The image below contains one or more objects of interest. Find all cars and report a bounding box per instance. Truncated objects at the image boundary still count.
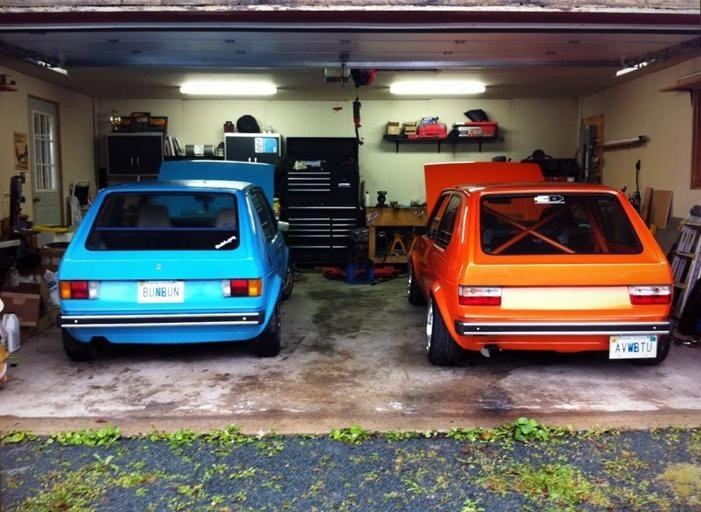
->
[407,159,679,367]
[54,160,298,359]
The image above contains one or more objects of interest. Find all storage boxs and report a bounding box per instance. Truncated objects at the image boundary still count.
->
[1,278,51,328]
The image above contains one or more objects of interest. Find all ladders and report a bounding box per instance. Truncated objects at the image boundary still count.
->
[668,215,701,328]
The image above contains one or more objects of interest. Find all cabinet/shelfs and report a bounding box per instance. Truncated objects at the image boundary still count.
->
[105,131,369,275]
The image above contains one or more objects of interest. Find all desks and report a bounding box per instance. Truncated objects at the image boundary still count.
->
[364,203,434,268]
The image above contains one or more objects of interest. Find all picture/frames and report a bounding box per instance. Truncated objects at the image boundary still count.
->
[116,110,169,135]
